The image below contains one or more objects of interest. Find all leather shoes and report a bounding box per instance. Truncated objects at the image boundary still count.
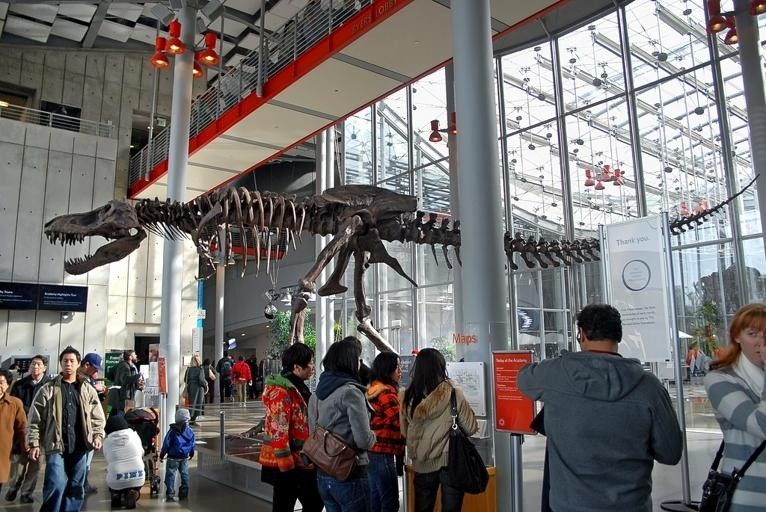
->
[4,488,34,503]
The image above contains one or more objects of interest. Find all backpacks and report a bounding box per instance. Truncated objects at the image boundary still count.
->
[107,361,131,381]
[220,359,232,376]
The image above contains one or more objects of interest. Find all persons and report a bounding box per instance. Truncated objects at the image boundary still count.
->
[703,303,766,512]
[184,350,262,425]
[400,348,478,512]
[517,304,683,512]
[1,345,145,512]
[158,409,195,503]
[258,343,324,512]
[308,336,403,512]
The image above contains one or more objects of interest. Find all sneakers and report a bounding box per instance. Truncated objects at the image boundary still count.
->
[111,490,137,510]
[166,492,188,500]
[192,421,200,427]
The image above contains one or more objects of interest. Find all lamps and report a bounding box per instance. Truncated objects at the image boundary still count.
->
[584,165,624,191]
[707,1,766,44]
[678,201,706,217]
[429,112,457,142]
[150,22,219,78]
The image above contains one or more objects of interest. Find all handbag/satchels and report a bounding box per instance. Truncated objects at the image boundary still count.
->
[699,468,738,512]
[441,424,489,494]
[208,364,216,381]
[182,384,189,399]
[234,372,241,378]
[302,425,356,481]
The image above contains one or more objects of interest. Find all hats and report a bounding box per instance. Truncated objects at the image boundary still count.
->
[175,409,190,423]
[83,353,101,369]
